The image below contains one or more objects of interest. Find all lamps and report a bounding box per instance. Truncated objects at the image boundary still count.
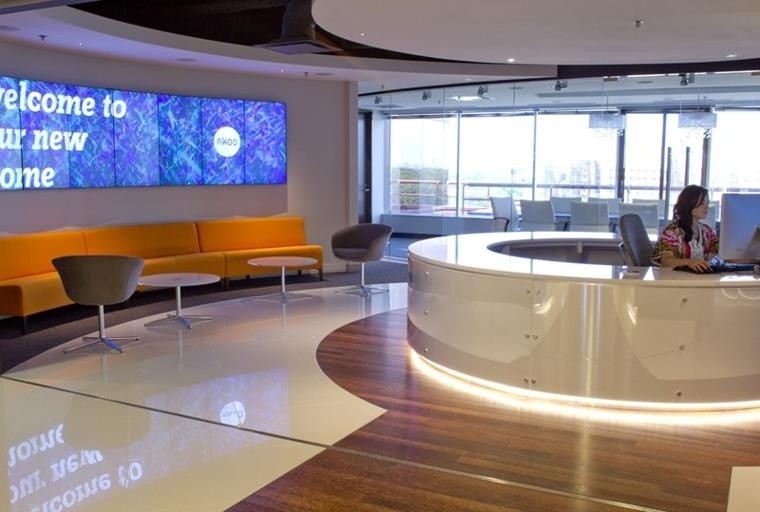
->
[552,79,571,93]
[589,80,625,137]
[476,83,490,98]
[677,74,697,87]
[421,89,432,102]
[678,94,716,139]
[372,95,384,104]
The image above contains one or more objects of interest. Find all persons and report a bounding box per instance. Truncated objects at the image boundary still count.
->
[649,185,718,274]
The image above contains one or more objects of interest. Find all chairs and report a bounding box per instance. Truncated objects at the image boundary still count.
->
[633,199,665,216]
[332,223,392,296]
[617,213,654,267]
[520,200,569,231]
[619,203,663,232]
[588,197,622,215]
[550,197,581,212]
[51,256,144,354]
[570,200,616,232]
[490,196,520,232]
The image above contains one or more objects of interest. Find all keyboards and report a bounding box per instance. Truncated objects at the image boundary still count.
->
[710,264,755,272]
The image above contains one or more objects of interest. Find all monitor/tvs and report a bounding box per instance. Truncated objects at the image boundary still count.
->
[718,193,760,275]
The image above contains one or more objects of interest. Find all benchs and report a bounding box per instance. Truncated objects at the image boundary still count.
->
[0,216,324,334]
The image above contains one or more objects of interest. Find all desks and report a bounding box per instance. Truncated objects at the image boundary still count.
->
[518,213,671,232]
